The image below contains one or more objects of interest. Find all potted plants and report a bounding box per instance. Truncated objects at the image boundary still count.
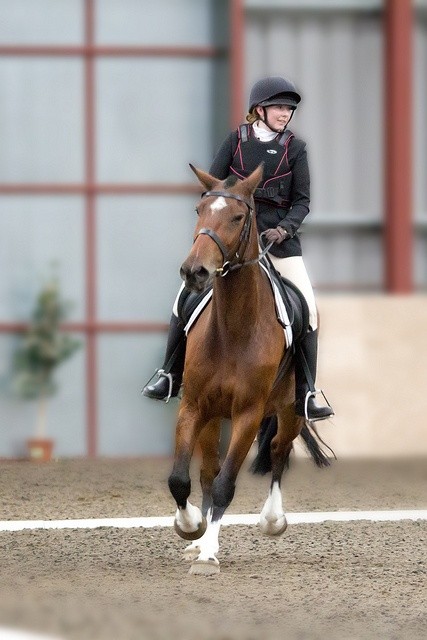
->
[9,282,83,464]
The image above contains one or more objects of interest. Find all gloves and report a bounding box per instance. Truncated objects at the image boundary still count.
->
[265,226,288,247]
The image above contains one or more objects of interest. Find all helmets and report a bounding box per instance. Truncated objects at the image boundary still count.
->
[249,77,301,114]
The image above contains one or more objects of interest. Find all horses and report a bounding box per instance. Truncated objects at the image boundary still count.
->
[167,159,336,576]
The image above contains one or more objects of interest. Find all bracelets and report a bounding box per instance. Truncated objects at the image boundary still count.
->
[276,225,286,238]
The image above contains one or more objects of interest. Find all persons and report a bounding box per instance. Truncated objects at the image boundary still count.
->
[141,77,334,420]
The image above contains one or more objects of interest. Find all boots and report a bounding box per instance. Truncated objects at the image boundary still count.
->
[295,329,333,417]
[143,312,186,399]
